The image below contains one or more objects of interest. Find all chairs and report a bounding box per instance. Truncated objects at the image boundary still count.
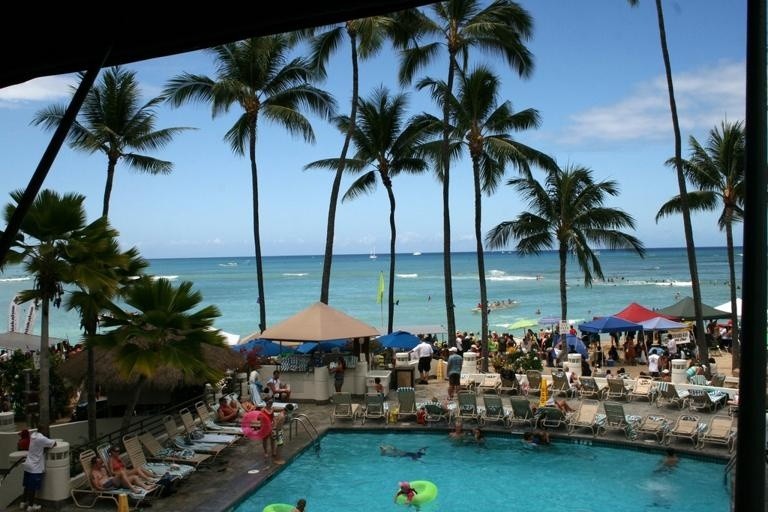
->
[209,402,263,431]
[123,435,193,483]
[98,444,162,497]
[248,387,298,420]
[140,429,209,472]
[70,449,149,506]
[220,393,283,434]
[178,407,241,450]
[193,400,252,441]
[163,416,228,460]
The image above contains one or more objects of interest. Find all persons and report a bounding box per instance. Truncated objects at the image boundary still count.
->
[373,321,740,395]
[523,399,576,449]
[417,396,491,449]
[216,370,295,464]
[291,499,305,512]
[326,356,345,392]
[19,422,57,512]
[477,297,514,314]
[380,443,431,465]
[90,457,157,494]
[107,445,158,483]
[393,479,420,512]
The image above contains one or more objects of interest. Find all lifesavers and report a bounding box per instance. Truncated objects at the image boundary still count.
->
[265,504,296,512]
[396,481,438,506]
[242,412,272,441]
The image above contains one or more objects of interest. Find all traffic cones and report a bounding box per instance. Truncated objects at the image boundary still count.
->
[118,493,129,512]
[539,378,548,406]
[436,358,445,381]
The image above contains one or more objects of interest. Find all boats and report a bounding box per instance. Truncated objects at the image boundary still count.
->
[471,296,521,317]
